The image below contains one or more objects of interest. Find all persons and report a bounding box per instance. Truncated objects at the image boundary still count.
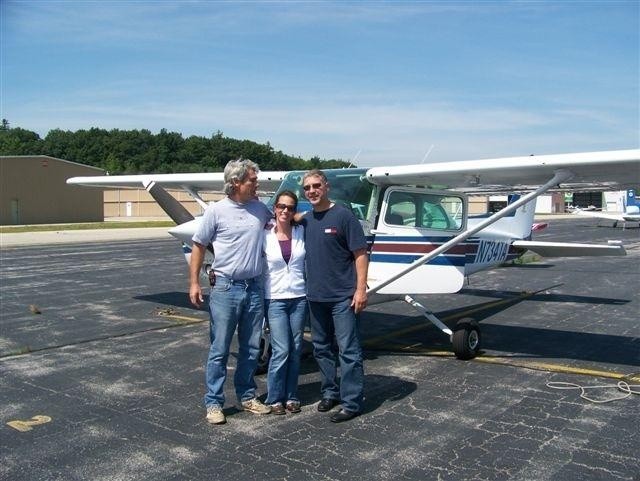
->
[304,169,369,423]
[189,158,277,425]
[258,190,307,416]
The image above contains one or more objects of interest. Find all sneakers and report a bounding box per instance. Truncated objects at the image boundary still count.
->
[236,397,271,414]
[206,406,226,424]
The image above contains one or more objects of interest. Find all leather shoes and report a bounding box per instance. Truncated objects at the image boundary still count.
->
[270,402,286,415]
[317,399,336,411]
[286,401,302,413]
[331,408,357,423]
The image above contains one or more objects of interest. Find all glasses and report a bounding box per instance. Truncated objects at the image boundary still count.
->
[275,204,296,212]
[303,184,321,190]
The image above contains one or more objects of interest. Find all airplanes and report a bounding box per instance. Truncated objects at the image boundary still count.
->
[65,147,639,374]
[573,190,639,230]
